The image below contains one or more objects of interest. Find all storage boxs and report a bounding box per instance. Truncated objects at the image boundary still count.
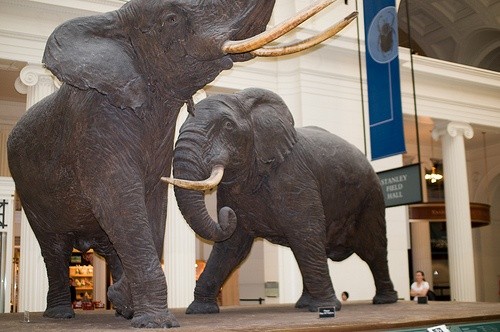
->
[73,301,104,311]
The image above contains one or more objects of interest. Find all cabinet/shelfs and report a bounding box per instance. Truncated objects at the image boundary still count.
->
[72,275,94,300]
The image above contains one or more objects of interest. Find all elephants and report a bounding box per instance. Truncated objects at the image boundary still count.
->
[160,87,398,314]
[6,0,359,327]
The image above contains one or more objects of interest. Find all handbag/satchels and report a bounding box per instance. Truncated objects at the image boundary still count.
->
[428,290,436,301]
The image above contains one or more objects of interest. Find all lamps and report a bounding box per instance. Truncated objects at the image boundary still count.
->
[424,129,443,183]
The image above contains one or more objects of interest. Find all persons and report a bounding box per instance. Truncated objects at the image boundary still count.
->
[342,291,349,301]
[69,277,76,305]
[410,271,429,301]
